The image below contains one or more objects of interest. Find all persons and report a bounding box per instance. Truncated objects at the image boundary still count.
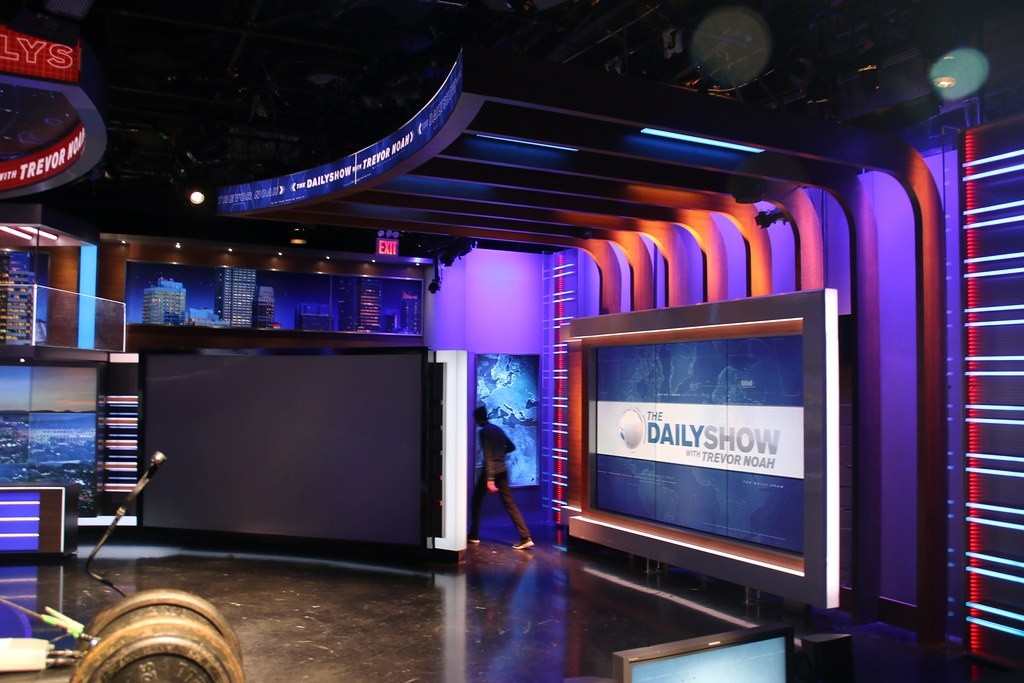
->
[470,407,533,550]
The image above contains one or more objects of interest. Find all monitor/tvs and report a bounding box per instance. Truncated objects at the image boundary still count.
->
[611,620,795,683]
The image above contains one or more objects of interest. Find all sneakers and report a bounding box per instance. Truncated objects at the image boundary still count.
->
[468,534,480,543]
[512,538,535,550]
